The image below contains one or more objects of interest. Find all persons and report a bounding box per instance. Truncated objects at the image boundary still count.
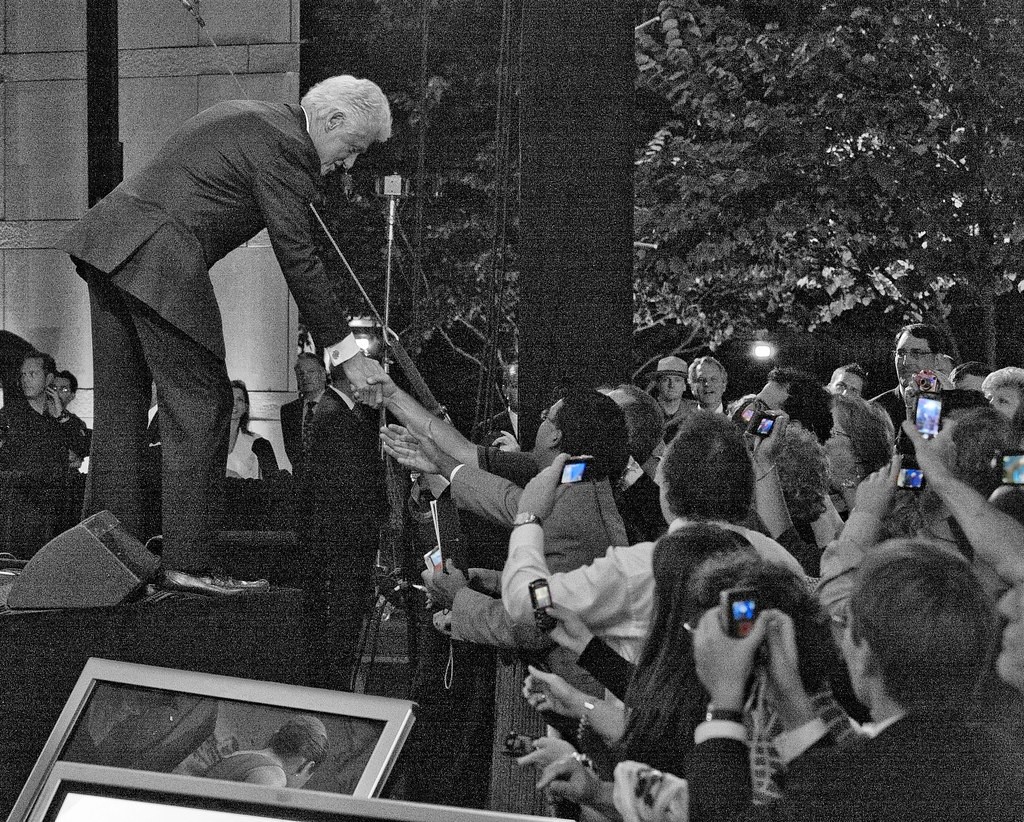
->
[203,716,327,788]
[0,350,92,559]
[350,325,1024,822]
[57,75,383,595]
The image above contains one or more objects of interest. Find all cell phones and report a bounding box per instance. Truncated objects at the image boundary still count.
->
[914,391,943,441]
[898,468,927,492]
[528,578,557,631]
[559,458,597,484]
[996,450,1024,486]
[720,588,758,639]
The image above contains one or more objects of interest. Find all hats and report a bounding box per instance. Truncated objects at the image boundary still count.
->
[648,355,689,379]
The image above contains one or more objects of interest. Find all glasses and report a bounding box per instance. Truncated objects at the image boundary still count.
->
[890,349,937,359]
[50,384,70,395]
[829,427,850,440]
[540,409,556,425]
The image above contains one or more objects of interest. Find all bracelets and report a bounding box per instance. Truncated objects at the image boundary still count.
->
[755,462,778,482]
[569,752,597,772]
[577,697,596,742]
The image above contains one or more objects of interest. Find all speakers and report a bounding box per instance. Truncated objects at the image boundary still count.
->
[5,510,162,608]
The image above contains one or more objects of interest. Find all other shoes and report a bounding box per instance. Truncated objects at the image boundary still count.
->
[161,567,270,597]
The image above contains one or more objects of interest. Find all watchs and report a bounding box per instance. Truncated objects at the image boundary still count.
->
[55,410,69,423]
[839,476,855,496]
[512,512,542,527]
[705,703,744,724]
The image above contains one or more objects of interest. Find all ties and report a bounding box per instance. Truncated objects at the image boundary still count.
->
[302,401,316,425]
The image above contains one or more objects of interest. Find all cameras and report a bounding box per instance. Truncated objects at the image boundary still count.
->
[747,409,777,440]
[423,546,441,572]
[920,374,941,393]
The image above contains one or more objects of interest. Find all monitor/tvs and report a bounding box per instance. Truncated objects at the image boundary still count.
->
[7,656,577,822]
